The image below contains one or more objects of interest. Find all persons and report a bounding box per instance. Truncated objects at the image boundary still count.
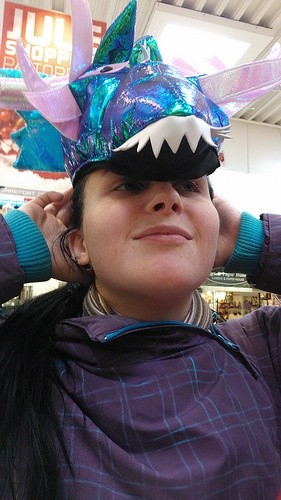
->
[1,0,281,500]
